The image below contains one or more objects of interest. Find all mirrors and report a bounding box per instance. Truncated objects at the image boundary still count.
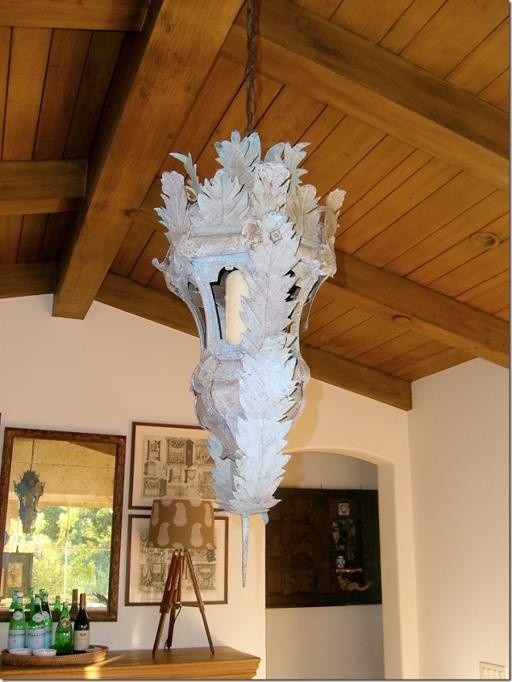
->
[0,427,127,622]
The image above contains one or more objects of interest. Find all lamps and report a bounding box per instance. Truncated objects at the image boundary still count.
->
[150,0,349,590]
[12,438,45,538]
[146,499,217,660]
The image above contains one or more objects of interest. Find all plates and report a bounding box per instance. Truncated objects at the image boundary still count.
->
[8,647,57,659]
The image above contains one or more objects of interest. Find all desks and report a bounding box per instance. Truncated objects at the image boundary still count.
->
[0,645,261,680]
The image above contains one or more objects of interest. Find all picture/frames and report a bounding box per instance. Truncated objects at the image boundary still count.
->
[127,421,225,512]
[2,552,33,598]
[125,513,228,607]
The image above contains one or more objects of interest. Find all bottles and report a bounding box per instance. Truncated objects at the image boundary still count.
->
[9,587,80,613]
[7,591,90,654]
